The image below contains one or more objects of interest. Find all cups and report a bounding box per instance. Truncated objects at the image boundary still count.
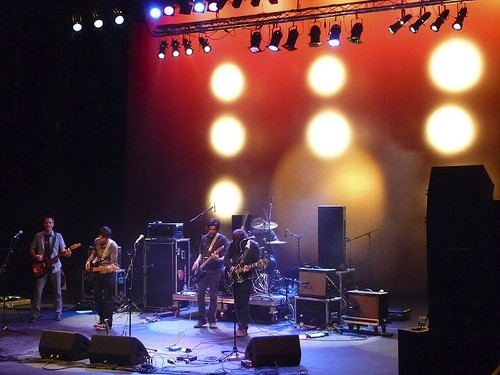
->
[419,317,427,330]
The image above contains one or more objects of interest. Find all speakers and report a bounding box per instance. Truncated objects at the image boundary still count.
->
[39,329,150,366]
[298,268,336,299]
[245,335,301,367]
[347,290,388,319]
[317,205,347,271]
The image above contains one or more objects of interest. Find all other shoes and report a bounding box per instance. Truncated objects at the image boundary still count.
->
[94,321,111,330]
[31,317,37,322]
[56,315,61,321]
[194,321,206,328]
[211,322,217,328]
[235,327,247,338]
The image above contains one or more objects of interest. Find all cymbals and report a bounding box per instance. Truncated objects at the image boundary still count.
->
[266,240,287,245]
[252,221,278,229]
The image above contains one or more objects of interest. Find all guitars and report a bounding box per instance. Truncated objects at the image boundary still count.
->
[225,258,267,287]
[190,245,224,283]
[31,243,81,279]
[82,264,120,281]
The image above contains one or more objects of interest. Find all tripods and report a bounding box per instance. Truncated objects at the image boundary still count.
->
[219,240,245,362]
[270,277,303,328]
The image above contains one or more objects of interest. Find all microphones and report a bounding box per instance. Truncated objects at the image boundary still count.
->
[14,230,23,238]
[284,229,288,237]
[243,236,256,241]
[303,281,309,284]
[142,264,154,268]
[349,305,358,308]
[270,197,272,208]
[214,203,215,212]
[134,234,144,243]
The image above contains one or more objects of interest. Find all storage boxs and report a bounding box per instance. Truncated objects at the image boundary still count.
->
[82,246,127,298]
[295,268,411,325]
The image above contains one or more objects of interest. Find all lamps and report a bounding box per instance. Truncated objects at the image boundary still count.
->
[72,0,469,59]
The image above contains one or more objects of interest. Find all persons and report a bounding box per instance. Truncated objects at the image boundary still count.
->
[29,215,72,323]
[193,218,228,329]
[85,226,119,330]
[249,220,279,292]
[224,228,260,337]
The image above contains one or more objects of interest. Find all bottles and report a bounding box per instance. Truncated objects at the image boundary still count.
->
[183,284,187,292]
